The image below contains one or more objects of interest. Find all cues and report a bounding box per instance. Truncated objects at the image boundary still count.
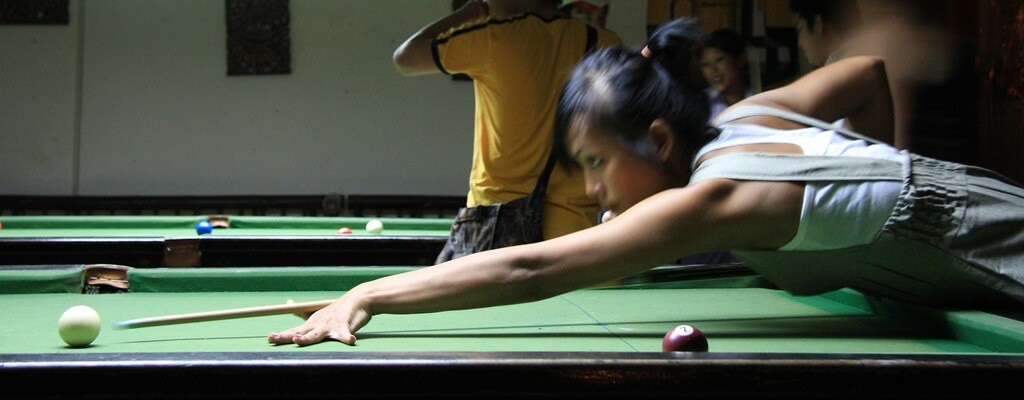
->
[113,264,753,331]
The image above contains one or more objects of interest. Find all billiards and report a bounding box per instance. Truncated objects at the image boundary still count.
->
[336,227,353,234]
[365,219,383,234]
[57,304,101,347]
[662,324,709,353]
[196,221,212,235]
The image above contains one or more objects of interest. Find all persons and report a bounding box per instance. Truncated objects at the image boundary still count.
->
[694,28,759,126]
[789,0,920,161]
[267,16,1024,346]
[392,0,625,288]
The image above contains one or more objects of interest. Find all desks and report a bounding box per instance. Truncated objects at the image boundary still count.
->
[0,215,456,265]
[0,263,1024,400]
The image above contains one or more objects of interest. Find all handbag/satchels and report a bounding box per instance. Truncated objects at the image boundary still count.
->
[450,197,544,265]
